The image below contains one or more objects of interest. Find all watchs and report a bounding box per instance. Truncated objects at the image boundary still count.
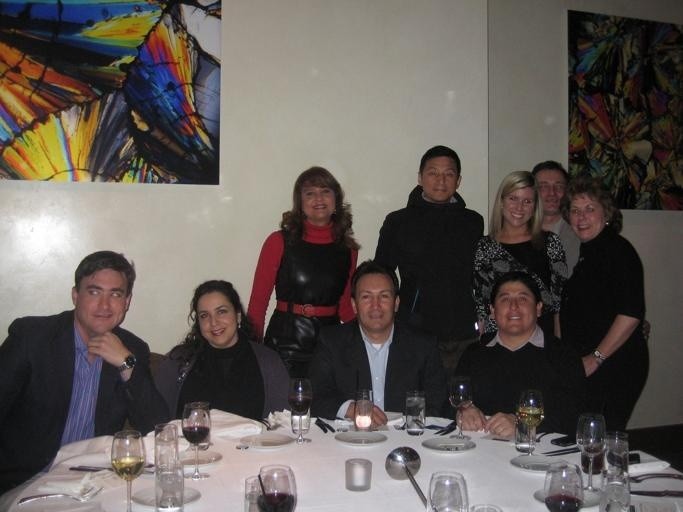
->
[116,352,138,373]
[593,349,609,361]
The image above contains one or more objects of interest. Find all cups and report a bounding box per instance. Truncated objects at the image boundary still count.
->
[447,376,472,439]
[183,402,210,478]
[545,462,583,512]
[354,389,373,430]
[518,391,546,457]
[427,471,466,512]
[289,397,310,436]
[154,467,185,510]
[575,416,603,491]
[258,465,297,512]
[407,392,425,436]
[345,458,372,492]
[598,484,632,512]
[603,431,632,496]
[110,431,146,512]
[288,379,310,445]
[513,412,540,453]
[244,477,261,511]
[154,424,178,484]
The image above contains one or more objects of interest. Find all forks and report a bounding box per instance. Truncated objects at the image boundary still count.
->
[17,486,106,506]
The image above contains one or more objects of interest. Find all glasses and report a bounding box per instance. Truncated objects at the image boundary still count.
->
[503,194,534,206]
[569,204,598,215]
[538,184,564,192]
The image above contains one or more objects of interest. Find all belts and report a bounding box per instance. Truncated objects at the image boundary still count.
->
[275,300,338,318]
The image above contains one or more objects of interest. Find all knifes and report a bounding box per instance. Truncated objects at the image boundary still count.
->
[631,490,683,498]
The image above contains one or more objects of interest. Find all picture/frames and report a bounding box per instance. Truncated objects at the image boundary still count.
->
[568,9,683,212]
[0,0,223,185]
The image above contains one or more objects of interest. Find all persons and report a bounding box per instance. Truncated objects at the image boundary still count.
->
[246,166,360,379]
[559,176,651,440]
[373,145,485,418]
[472,170,569,342]
[530,160,582,341]
[0,250,172,496]
[164,279,292,423]
[308,261,448,432]
[448,270,586,442]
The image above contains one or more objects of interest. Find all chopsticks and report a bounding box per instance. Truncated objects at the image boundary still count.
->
[70,465,154,478]
[544,447,577,456]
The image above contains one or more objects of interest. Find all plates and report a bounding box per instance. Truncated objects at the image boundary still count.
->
[533,486,603,507]
[334,432,387,445]
[511,455,567,472]
[177,449,222,466]
[240,433,293,448]
[132,486,202,507]
[421,438,476,452]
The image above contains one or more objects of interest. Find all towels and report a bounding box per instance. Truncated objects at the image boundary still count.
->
[147,407,263,441]
[603,447,671,476]
